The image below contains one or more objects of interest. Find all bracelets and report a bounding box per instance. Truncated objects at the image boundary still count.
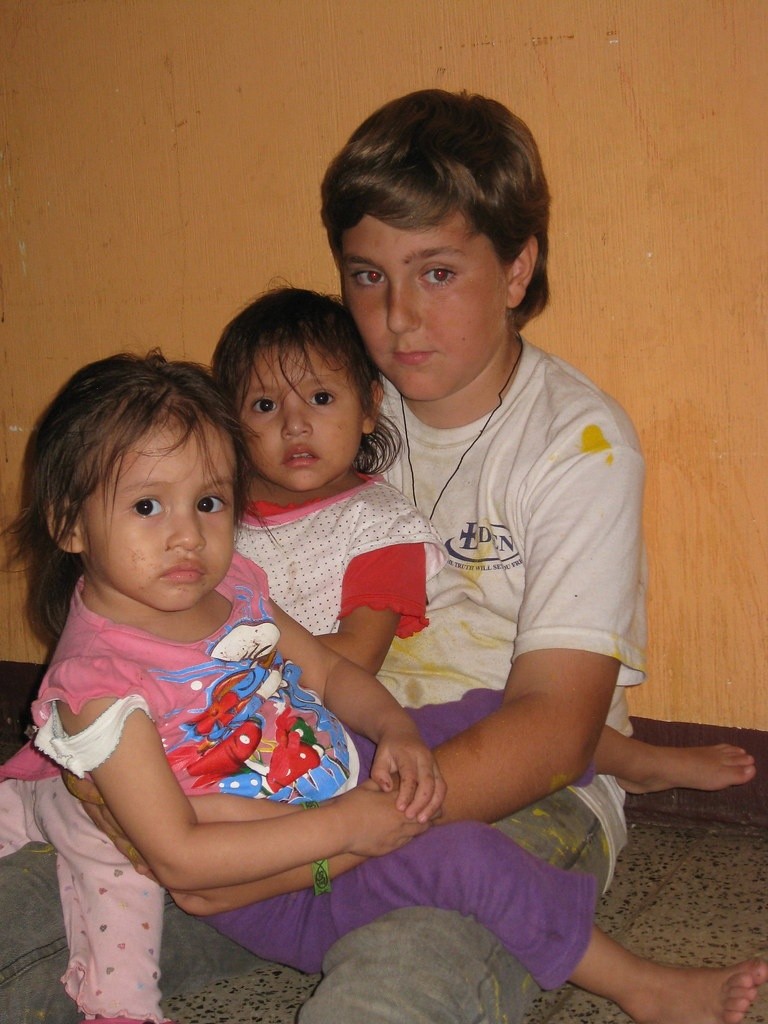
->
[302,799,333,895]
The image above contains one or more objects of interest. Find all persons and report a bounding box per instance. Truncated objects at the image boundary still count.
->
[1,87,649,1024]
[2,348,767,1022]
[2,282,431,1024]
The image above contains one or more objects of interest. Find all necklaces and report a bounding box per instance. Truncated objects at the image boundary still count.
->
[395,330,525,521]
